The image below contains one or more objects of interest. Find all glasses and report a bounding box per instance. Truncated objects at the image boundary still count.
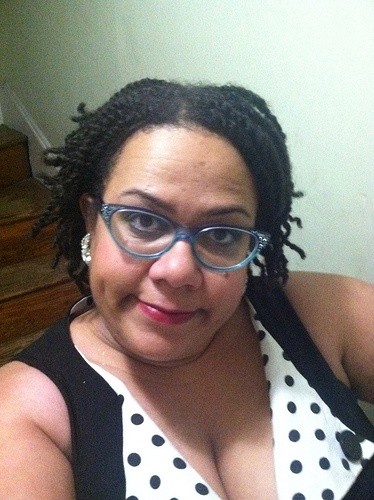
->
[91,194,269,273]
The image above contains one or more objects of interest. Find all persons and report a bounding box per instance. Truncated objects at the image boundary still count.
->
[2,76,373,499]
